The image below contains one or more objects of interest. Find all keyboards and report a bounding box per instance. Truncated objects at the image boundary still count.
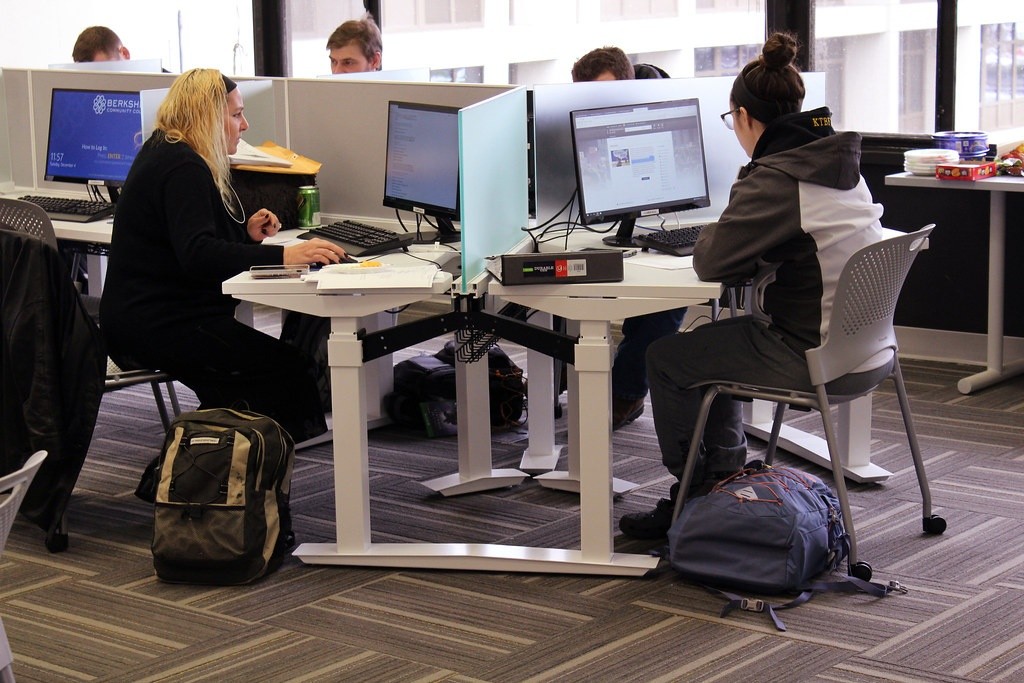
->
[296,220,415,257]
[634,225,707,258]
[16,195,116,223]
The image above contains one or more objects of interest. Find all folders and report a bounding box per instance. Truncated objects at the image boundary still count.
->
[484,250,624,287]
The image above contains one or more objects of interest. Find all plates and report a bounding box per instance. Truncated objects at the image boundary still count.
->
[904,149,959,175]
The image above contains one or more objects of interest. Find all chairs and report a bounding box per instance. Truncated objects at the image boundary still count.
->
[0,449,49,682]
[0,199,183,552]
[670,224,947,584]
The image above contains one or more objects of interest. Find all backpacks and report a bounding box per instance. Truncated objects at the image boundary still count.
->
[136,404,295,590]
[665,461,848,613]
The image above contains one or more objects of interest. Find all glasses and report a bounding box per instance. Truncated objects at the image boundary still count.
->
[720,107,743,131]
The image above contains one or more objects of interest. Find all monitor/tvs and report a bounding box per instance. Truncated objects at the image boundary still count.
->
[382,100,461,244]
[44,88,144,204]
[569,97,711,248]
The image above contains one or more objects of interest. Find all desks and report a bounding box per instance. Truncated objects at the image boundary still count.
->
[885,170,1024,394]
[2,184,892,580]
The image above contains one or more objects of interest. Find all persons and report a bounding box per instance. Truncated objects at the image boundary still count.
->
[326,19,382,73]
[72,26,130,295]
[522,47,685,424]
[98,69,349,502]
[621,32,895,538]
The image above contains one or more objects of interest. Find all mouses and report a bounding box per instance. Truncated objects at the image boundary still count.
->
[312,255,358,266]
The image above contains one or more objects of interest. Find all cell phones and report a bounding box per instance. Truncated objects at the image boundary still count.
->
[250,264,310,276]
[579,247,636,258]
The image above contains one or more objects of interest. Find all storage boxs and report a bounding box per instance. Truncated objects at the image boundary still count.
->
[936,159,997,180]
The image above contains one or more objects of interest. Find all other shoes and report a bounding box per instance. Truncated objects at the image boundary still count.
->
[612,400,645,428]
[618,484,687,541]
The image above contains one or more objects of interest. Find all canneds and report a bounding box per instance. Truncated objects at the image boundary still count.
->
[298,185,321,230]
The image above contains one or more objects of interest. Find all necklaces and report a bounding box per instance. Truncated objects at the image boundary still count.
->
[224,184,245,224]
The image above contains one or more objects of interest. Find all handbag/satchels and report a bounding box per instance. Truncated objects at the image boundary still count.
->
[387,341,525,438]
